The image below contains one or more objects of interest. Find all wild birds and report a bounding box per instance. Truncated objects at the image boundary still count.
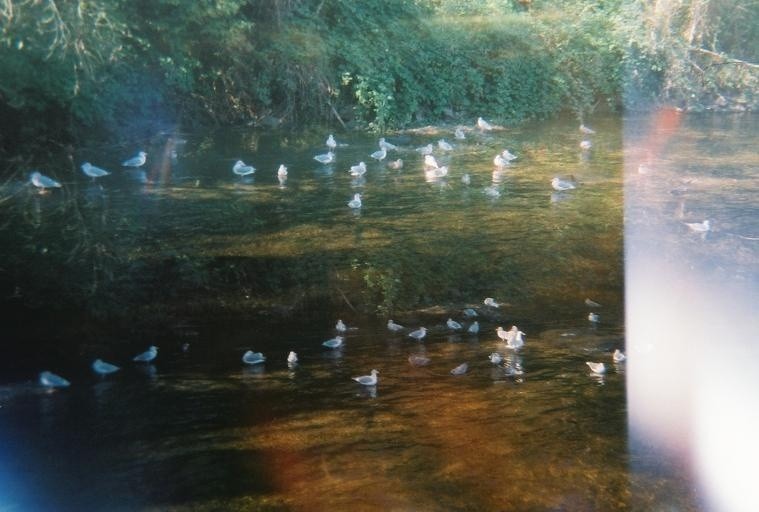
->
[232,117,595,209]
[80,163,112,177]
[134,345,159,361]
[93,359,120,375]
[122,151,148,167]
[39,371,70,394]
[30,171,61,195]
[638,164,651,177]
[685,220,711,232]
[242,297,626,385]
